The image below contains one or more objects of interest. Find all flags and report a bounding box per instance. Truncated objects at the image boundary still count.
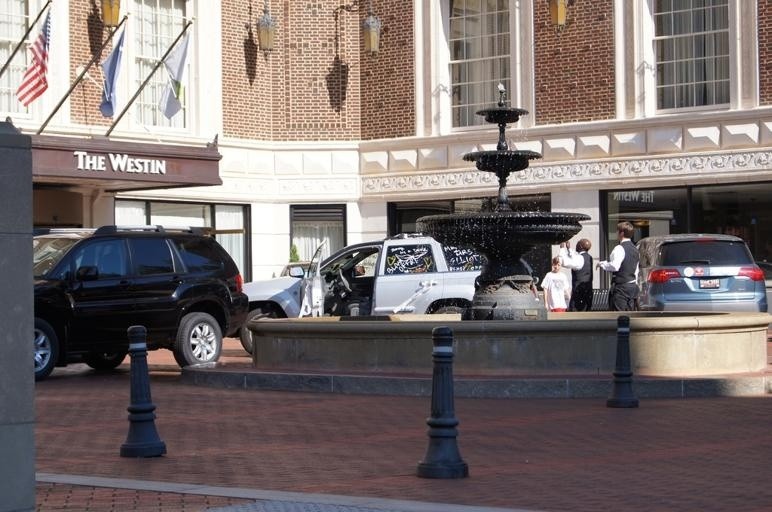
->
[158,26,191,120]
[15,8,51,107]
[99,24,126,117]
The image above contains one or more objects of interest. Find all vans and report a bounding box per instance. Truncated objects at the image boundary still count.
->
[632,230,770,316]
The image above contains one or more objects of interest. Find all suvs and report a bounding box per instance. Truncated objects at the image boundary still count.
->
[33,225,252,382]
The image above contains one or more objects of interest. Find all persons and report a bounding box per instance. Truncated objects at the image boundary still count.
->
[595,222,640,311]
[541,239,593,311]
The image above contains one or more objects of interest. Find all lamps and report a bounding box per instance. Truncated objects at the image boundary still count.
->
[548,0,575,24]
[331,0,382,54]
[245,1,273,50]
[89,0,119,28]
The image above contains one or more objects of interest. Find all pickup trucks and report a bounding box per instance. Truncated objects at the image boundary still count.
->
[241,229,481,356]
